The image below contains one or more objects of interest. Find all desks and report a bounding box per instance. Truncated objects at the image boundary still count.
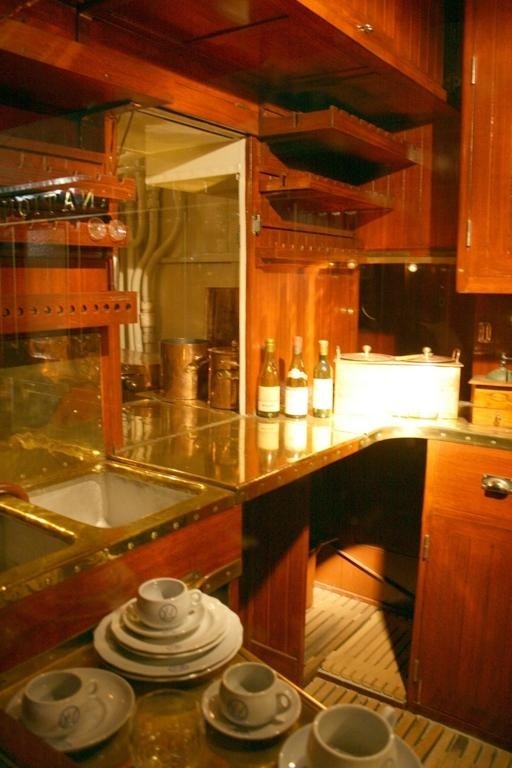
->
[0,640,374,767]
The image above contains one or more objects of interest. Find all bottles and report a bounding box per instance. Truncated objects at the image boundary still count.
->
[256,419,279,475]
[310,421,332,454]
[286,335,309,419]
[313,340,333,419]
[284,421,307,466]
[256,337,281,418]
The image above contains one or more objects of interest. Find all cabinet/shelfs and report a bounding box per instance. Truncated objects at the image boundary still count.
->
[254,107,415,417]
[453,2,510,296]
[0,476,311,739]
[406,421,511,754]
[131,1,448,130]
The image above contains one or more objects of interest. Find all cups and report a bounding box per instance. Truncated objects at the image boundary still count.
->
[308,703,398,768]
[22,670,99,739]
[220,662,294,727]
[137,577,203,629]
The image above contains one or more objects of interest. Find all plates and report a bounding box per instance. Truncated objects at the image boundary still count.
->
[278,718,425,768]
[93,591,244,683]
[5,667,136,754]
[110,600,228,662]
[201,677,302,741]
[123,601,207,639]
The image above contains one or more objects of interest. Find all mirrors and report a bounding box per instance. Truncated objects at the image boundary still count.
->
[110,109,246,492]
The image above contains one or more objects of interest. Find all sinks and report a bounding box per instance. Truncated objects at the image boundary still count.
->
[0,502,77,576]
[0,429,100,494]
[16,459,208,531]
